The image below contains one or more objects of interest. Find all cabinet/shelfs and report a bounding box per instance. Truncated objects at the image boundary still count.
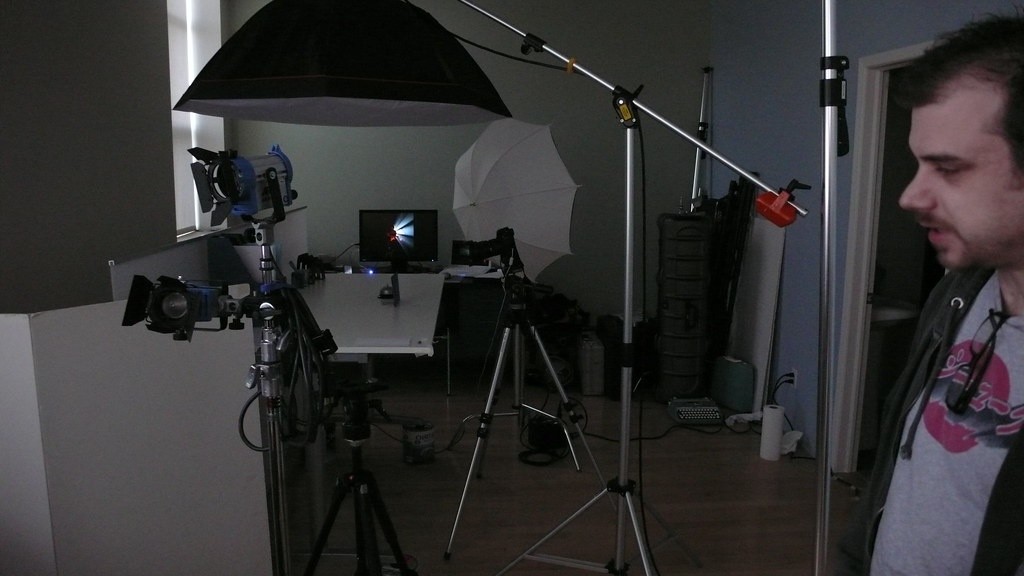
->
[326,265,513,365]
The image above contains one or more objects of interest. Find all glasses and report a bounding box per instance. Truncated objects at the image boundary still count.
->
[945,307,1013,413]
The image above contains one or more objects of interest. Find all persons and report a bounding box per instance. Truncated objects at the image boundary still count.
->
[835,15,1024,576]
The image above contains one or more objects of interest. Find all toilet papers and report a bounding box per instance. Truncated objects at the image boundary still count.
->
[760,405,785,461]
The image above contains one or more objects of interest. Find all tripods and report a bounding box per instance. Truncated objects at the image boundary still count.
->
[300,0,808,576]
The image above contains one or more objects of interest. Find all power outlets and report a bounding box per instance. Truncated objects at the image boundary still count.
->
[791,368,799,389]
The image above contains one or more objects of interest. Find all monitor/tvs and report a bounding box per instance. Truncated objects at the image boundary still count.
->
[358,210,439,273]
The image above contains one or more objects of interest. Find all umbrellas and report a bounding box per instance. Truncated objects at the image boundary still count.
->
[451,120,582,281]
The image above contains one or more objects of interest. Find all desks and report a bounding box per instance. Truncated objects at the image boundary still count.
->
[283,269,446,428]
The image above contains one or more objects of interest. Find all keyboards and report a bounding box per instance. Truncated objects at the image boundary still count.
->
[677,408,720,419]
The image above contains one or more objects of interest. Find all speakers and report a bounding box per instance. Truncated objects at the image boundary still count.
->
[655,212,714,402]
